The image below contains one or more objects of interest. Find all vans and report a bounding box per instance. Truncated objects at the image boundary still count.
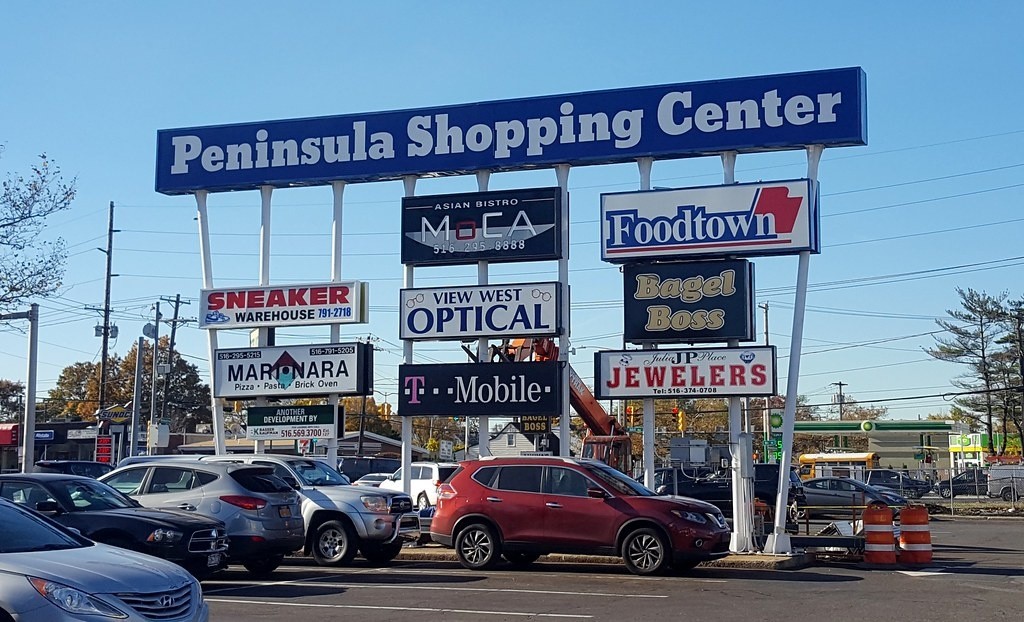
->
[985,464,1024,503]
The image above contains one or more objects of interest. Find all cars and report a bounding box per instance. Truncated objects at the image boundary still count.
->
[0,495,210,622]
[933,468,989,500]
[800,475,910,520]
[864,467,932,500]
[351,472,395,490]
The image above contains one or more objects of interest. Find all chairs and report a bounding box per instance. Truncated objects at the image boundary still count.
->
[28,487,47,508]
[559,475,572,495]
[836,481,844,490]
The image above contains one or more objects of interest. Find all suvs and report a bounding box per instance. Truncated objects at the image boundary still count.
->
[105,455,423,567]
[429,455,732,576]
[336,454,416,489]
[653,462,808,524]
[36,459,115,481]
[44,460,306,581]
[634,466,714,491]
[378,462,459,512]
[0,472,232,584]
